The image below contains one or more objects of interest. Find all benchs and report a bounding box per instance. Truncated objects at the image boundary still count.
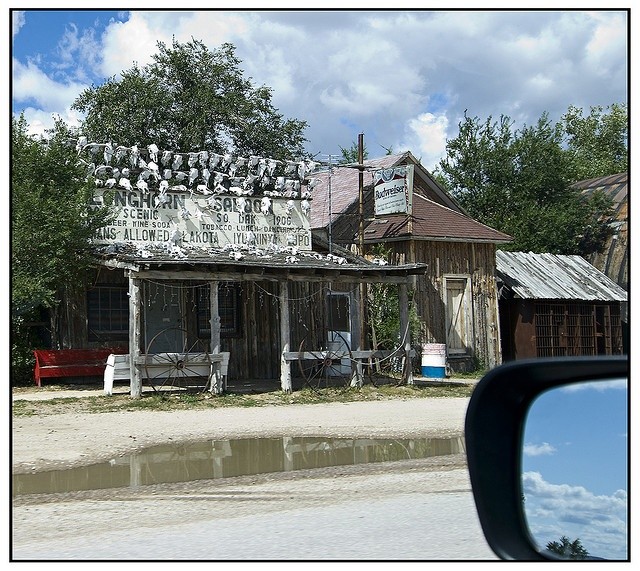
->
[32,347,129,388]
[104,351,231,398]
